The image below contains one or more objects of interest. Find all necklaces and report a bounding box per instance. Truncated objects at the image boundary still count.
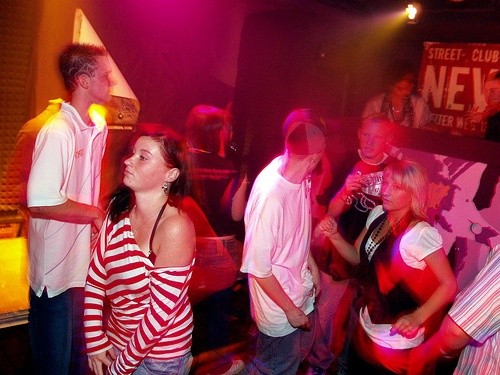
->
[130,205,170,234]
[365,215,406,259]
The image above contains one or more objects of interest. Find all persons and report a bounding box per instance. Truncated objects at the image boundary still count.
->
[25,44,500,375]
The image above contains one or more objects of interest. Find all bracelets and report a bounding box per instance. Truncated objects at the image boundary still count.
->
[338,192,347,202]
